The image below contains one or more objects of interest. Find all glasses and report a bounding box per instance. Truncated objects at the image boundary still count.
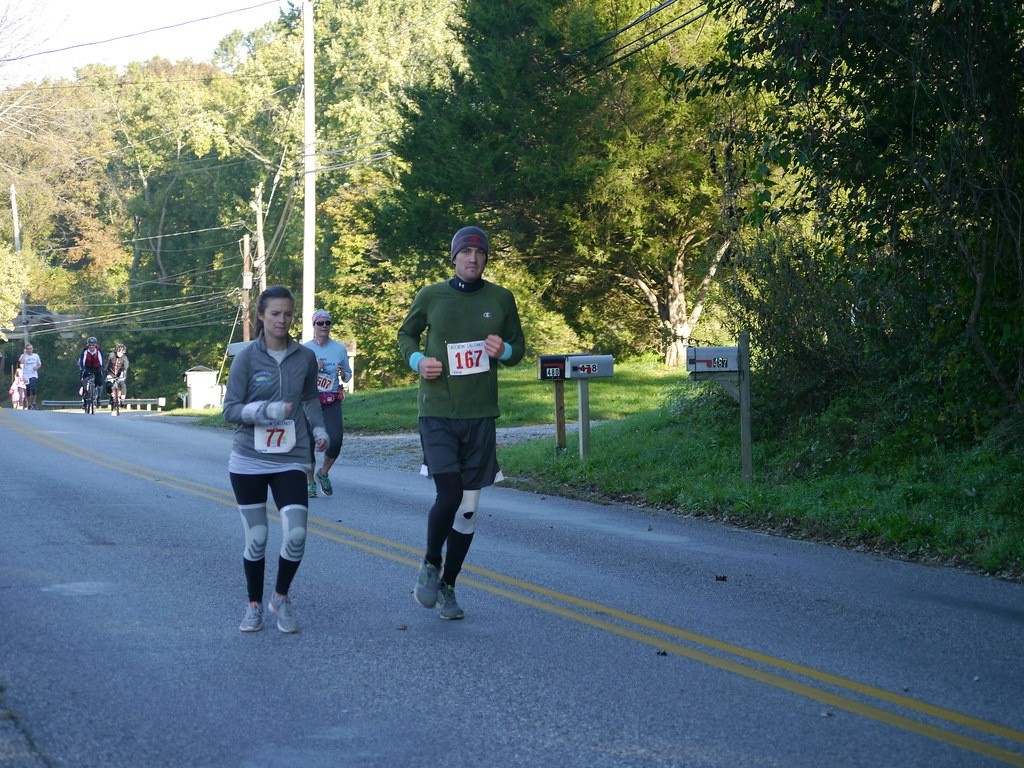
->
[314,320,331,326]
[88,344,95,347]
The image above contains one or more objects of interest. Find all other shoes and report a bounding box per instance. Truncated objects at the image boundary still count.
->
[121,400,126,406]
[82,401,86,408]
[109,396,113,404]
[97,397,102,406]
[28,403,39,410]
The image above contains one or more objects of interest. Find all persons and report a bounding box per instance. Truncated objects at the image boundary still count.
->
[9,343,42,411]
[301,309,352,496]
[223,285,329,634]
[103,343,129,407]
[79,337,103,409]
[397,226,525,618]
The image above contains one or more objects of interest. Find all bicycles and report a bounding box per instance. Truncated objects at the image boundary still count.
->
[108,376,123,417]
[80,369,97,414]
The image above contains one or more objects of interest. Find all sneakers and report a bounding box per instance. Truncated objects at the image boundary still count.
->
[306,480,317,498]
[437,577,463,620]
[315,467,333,496]
[239,603,264,631]
[268,592,297,632]
[413,557,441,609]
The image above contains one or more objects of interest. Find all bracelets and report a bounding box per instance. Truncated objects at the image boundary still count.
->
[410,352,424,372]
[499,342,511,362]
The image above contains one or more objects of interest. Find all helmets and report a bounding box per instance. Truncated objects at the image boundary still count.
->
[87,337,97,343]
[116,344,127,353]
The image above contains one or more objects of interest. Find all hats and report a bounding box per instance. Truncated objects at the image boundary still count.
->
[451,228,488,268]
